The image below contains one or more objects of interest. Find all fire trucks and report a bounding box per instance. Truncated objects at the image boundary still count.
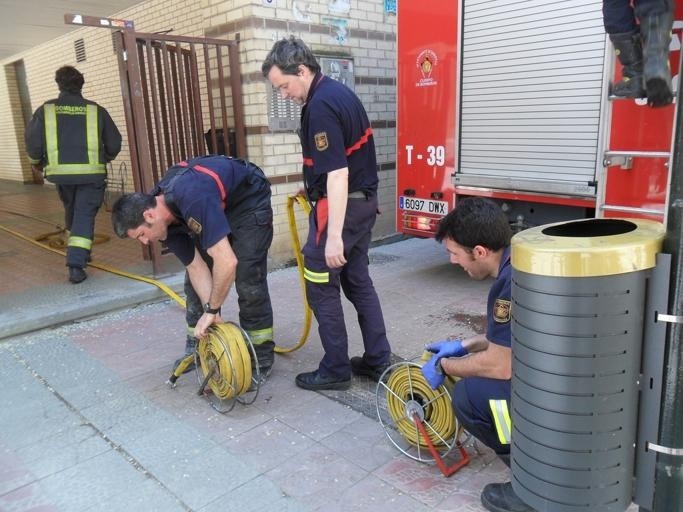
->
[395,2,682,241]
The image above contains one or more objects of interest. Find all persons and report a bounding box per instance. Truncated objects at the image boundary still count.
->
[25,66,121,283]
[112,154,275,392]
[262,35,392,389]
[603,0,674,107]
[425,199,537,512]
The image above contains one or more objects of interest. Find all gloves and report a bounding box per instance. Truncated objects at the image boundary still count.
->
[420,355,448,391]
[426,337,469,368]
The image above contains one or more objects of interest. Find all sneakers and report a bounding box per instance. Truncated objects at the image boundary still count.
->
[350,354,392,383]
[68,265,88,284]
[294,369,352,392]
[612,78,647,97]
[480,480,538,512]
[173,354,203,375]
[644,77,674,108]
[248,365,274,392]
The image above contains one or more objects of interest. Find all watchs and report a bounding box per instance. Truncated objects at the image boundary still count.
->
[203,303,220,315]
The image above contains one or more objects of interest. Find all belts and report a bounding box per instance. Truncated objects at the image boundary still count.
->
[347,189,367,200]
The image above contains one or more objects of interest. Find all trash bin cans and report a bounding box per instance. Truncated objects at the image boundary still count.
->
[510,217,672,512]
